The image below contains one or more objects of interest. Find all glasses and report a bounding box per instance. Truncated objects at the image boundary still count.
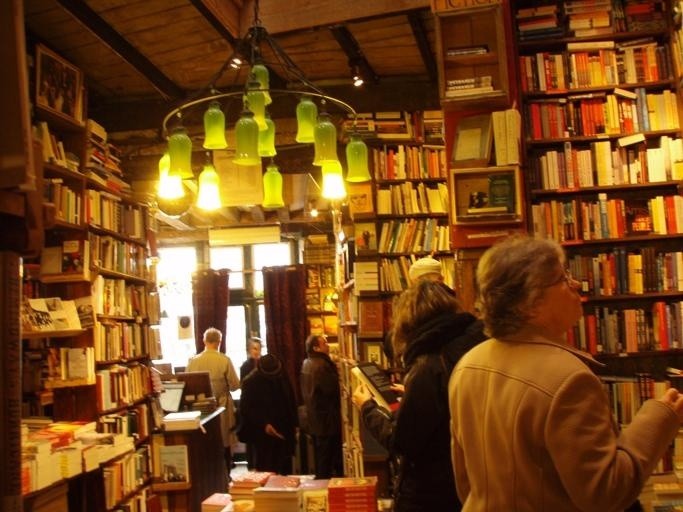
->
[545,268,573,288]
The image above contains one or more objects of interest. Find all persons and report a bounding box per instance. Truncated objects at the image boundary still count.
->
[186,328,238,460]
[240,336,262,381]
[408,259,444,286]
[237,354,295,475]
[299,334,344,480]
[352,279,489,511]
[449,235,683,510]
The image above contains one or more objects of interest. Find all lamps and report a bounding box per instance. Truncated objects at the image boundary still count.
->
[156,25,372,212]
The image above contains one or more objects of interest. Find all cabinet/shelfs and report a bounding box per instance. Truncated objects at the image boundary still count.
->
[430,0,682,512]
[1,2,169,512]
[332,104,455,480]
[299,225,339,370]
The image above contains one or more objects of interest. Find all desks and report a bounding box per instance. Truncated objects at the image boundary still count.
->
[162,402,231,511]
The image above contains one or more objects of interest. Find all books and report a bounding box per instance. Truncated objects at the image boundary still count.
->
[302,234,336,332]
[201,470,380,512]
[530,87,679,140]
[532,193,683,240]
[98,401,161,443]
[600,367,682,430]
[568,302,682,354]
[371,146,446,179]
[540,134,683,190]
[378,219,450,252]
[518,32,682,92]
[445,44,492,54]
[38,348,96,386]
[445,75,495,94]
[41,240,89,274]
[21,417,97,495]
[103,444,148,512]
[75,321,161,359]
[380,256,454,290]
[99,237,149,278]
[514,1,682,38]
[42,121,145,241]
[377,183,448,213]
[568,251,681,295]
[25,297,81,330]
[99,363,166,411]
[95,276,160,319]
[453,109,522,168]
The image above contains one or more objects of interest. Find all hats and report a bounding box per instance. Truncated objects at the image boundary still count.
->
[409,257,441,281]
[256,355,283,377]
